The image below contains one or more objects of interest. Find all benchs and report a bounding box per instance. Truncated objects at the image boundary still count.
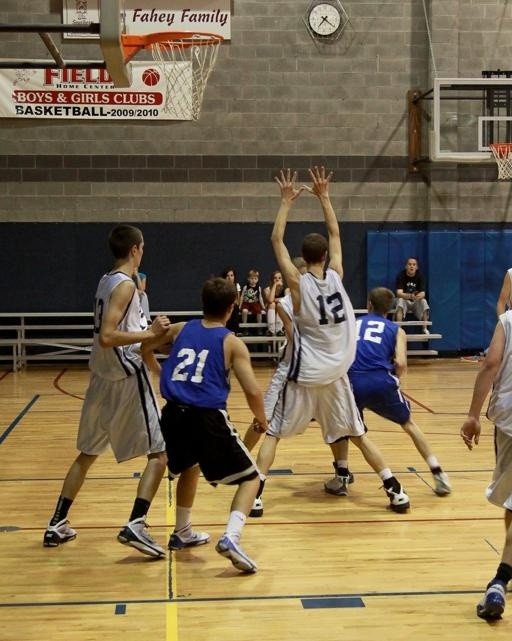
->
[0,311,441,371]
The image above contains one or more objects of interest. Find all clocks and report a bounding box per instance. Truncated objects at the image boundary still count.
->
[300,1,351,40]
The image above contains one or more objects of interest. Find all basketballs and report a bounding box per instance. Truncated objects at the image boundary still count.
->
[143,68,160,85]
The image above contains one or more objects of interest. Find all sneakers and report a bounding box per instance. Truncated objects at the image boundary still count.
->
[257,328,263,335]
[432,472,452,494]
[383,483,410,511]
[116,516,167,559]
[242,329,250,336]
[324,463,355,497]
[265,329,286,336]
[215,531,258,573]
[422,328,430,334]
[476,578,507,620]
[249,496,264,517]
[43,516,77,548]
[168,522,212,550]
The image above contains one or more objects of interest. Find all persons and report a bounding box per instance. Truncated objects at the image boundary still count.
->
[496,267,512,322]
[460,309,512,624]
